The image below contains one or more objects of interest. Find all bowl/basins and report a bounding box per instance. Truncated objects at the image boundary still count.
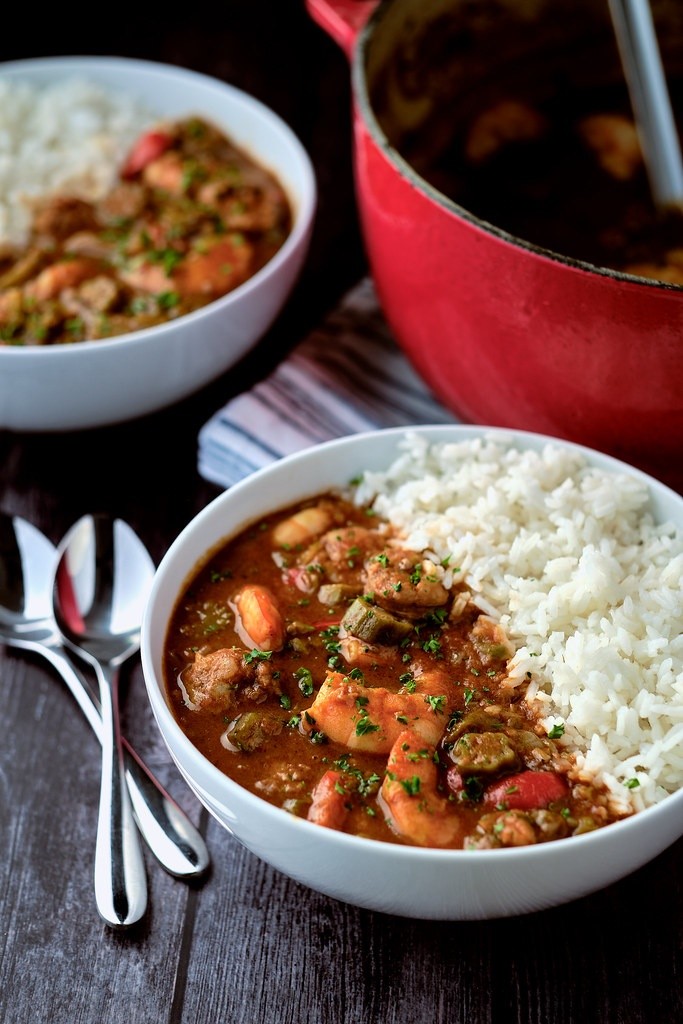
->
[142,422,683,920]
[1,58,314,429]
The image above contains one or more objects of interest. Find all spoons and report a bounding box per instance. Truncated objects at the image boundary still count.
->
[51,511,155,929]
[1,511,209,879]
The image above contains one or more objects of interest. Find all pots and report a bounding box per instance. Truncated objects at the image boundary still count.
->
[306,0,682,501]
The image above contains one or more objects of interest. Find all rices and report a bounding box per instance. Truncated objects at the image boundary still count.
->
[344,434,683,823]
